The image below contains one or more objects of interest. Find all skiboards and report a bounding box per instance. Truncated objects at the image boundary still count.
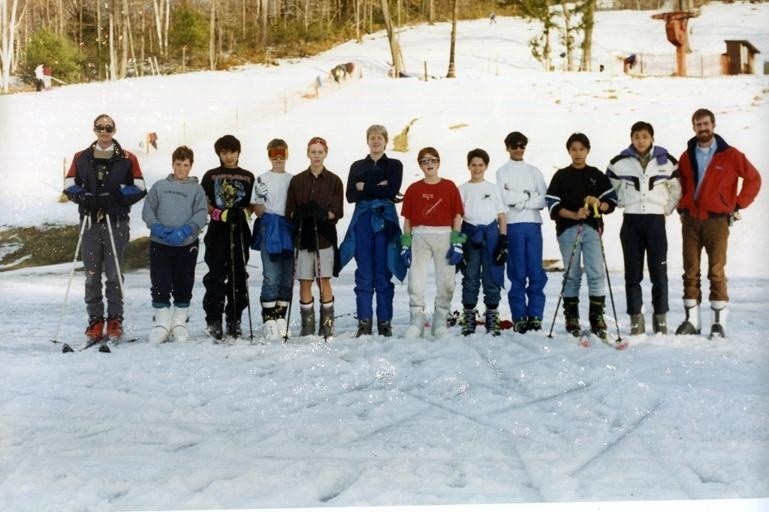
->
[61,338,115,352]
[573,335,628,349]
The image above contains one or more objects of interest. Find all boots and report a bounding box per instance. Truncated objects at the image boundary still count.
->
[564,295,582,335]
[626,311,646,339]
[527,312,544,332]
[484,307,503,338]
[71,295,453,343]
[510,315,528,334]
[706,297,730,342]
[586,296,608,331]
[649,311,668,336]
[462,306,475,336]
[674,296,704,338]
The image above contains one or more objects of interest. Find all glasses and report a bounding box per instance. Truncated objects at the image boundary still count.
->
[508,143,528,151]
[94,124,115,133]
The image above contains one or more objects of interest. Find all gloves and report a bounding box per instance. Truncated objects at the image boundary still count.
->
[399,246,413,269]
[231,208,254,225]
[165,223,194,247]
[210,206,235,222]
[150,222,173,241]
[76,190,97,209]
[252,182,268,205]
[445,244,512,267]
[96,191,117,211]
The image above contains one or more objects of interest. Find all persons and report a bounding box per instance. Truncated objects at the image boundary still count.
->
[337,124,407,339]
[545,132,619,338]
[495,130,548,334]
[34,63,52,92]
[457,148,509,339]
[488,10,497,25]
[140,145,209,344]
[605,120,684,337]
[201,134,344,344]
[63,112,148,344]
[400,147,467,342]
[674,108,762,339]
